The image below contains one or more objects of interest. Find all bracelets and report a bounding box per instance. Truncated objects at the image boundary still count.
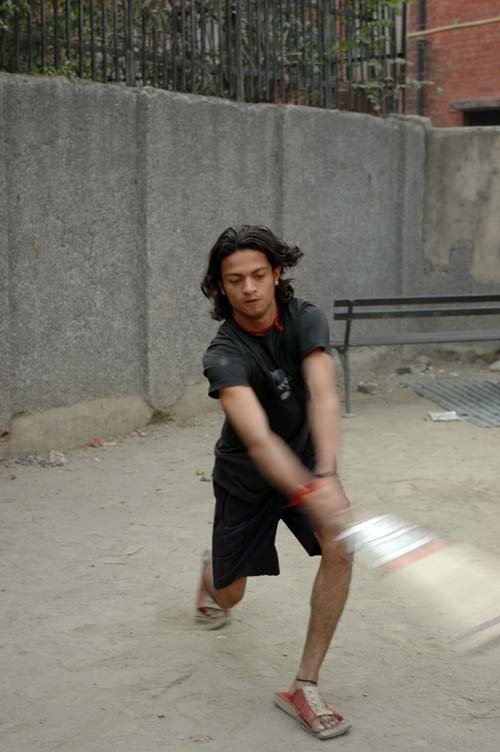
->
[313,471,336,480]
[287,482,320,509]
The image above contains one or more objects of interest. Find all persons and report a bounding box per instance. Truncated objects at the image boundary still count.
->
[192,224,356,740]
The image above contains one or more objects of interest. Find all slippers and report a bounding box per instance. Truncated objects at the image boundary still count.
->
[274,685,352,740]
[194,550,229,630]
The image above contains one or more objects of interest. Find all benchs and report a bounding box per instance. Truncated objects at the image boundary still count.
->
[328,293,500,412]
